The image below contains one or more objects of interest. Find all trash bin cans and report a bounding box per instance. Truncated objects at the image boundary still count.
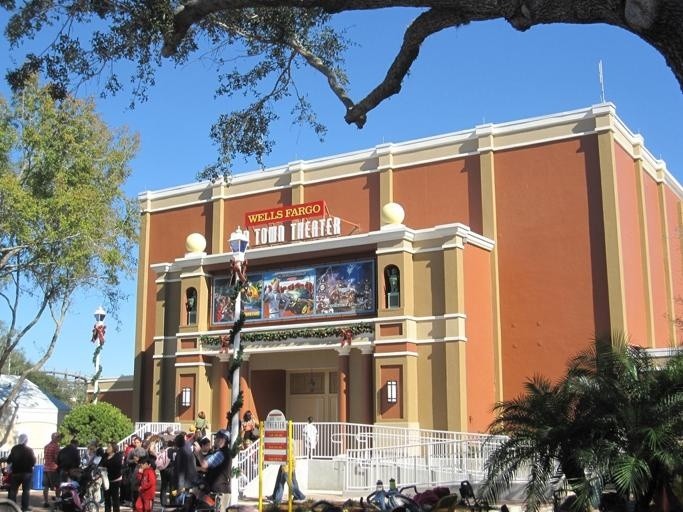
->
[35,465,44,490]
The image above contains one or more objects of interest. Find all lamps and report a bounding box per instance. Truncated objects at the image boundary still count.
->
[385,379,396,404]
[181,387,191,407]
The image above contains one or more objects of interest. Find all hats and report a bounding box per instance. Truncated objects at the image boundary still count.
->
[211,429,231,441]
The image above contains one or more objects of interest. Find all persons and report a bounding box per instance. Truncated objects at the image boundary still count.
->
[302,416,318,449]
[263,440,306,504]
[0,410,259,512]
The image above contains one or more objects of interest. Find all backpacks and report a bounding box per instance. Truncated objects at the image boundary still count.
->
[147,440,172,471]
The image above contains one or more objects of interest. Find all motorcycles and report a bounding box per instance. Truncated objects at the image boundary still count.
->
[48,455,104,512]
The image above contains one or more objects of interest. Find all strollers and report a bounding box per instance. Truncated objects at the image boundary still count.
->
[156,448,207,512]
[0,456,15,491]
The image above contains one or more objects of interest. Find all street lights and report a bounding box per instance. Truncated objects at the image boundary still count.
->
[226,224,252,507]
[89,302,108,405]
